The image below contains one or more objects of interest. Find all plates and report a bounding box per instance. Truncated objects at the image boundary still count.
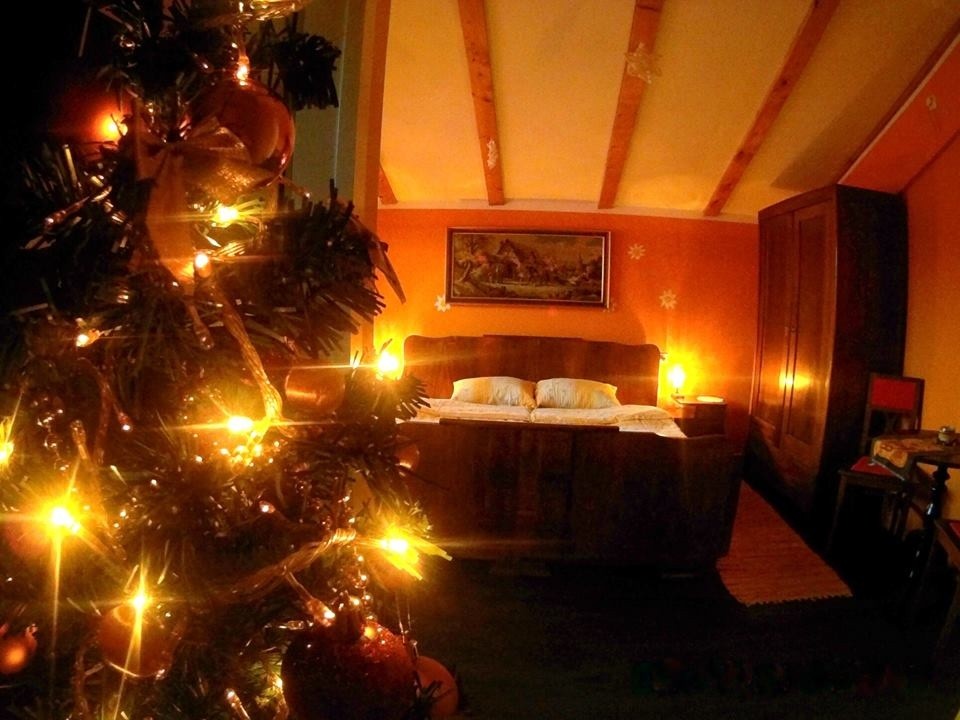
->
[698,396,723,403]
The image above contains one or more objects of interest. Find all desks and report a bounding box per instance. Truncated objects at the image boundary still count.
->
[872,430,960,584]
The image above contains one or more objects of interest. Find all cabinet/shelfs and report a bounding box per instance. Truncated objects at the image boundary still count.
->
[756,184,908,507]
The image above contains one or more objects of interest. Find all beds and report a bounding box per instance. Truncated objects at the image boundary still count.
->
[394,334,741,571]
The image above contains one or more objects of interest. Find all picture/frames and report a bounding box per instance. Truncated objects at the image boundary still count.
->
[446,228,610,308]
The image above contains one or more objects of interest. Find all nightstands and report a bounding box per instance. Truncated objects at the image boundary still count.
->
[676,397,727,436]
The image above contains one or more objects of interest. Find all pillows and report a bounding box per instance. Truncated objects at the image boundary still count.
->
[535,378,622,407]
[452,375,536,408]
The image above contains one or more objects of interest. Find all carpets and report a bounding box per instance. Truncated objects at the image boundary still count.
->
[715,479,853,607]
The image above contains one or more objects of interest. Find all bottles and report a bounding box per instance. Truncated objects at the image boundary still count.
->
[938,425,956,444]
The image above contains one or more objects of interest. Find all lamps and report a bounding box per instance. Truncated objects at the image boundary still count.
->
[668,365,686,399]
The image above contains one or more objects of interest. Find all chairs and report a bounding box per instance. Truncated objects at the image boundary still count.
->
[837,375,924,533]
